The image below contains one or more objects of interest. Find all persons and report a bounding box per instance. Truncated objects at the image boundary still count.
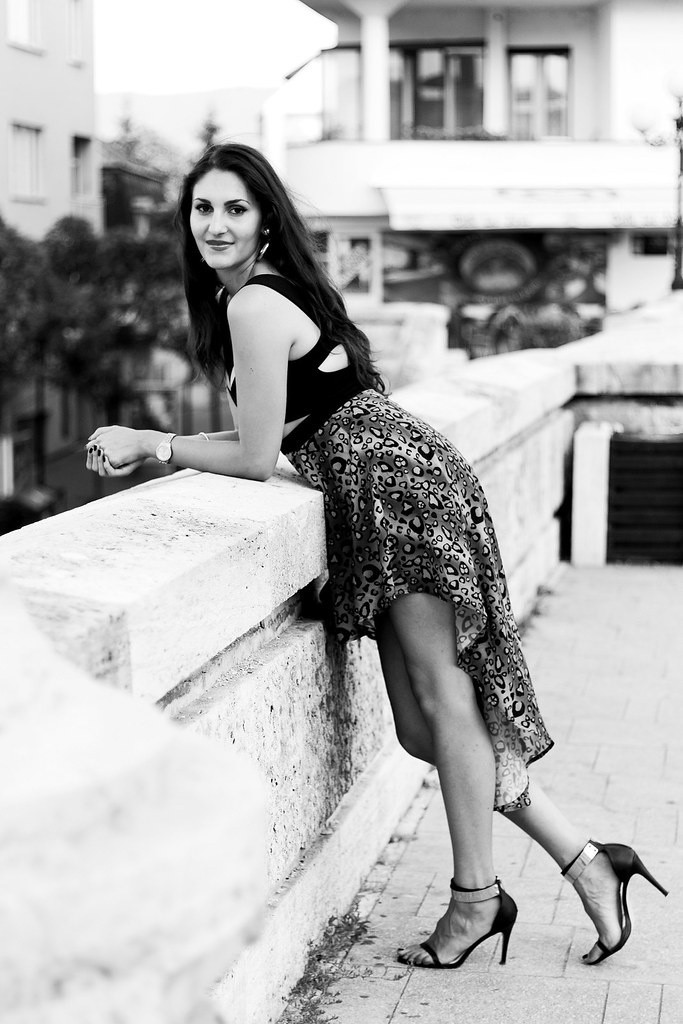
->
[86,142,669,970]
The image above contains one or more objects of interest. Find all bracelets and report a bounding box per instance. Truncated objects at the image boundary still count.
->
[199,432,210,440]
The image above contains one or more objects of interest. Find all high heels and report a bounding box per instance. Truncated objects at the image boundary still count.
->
[560,838,668,966]
[397,876,518,969]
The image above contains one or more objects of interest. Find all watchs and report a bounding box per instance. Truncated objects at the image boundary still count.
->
[156,433,177,464]
